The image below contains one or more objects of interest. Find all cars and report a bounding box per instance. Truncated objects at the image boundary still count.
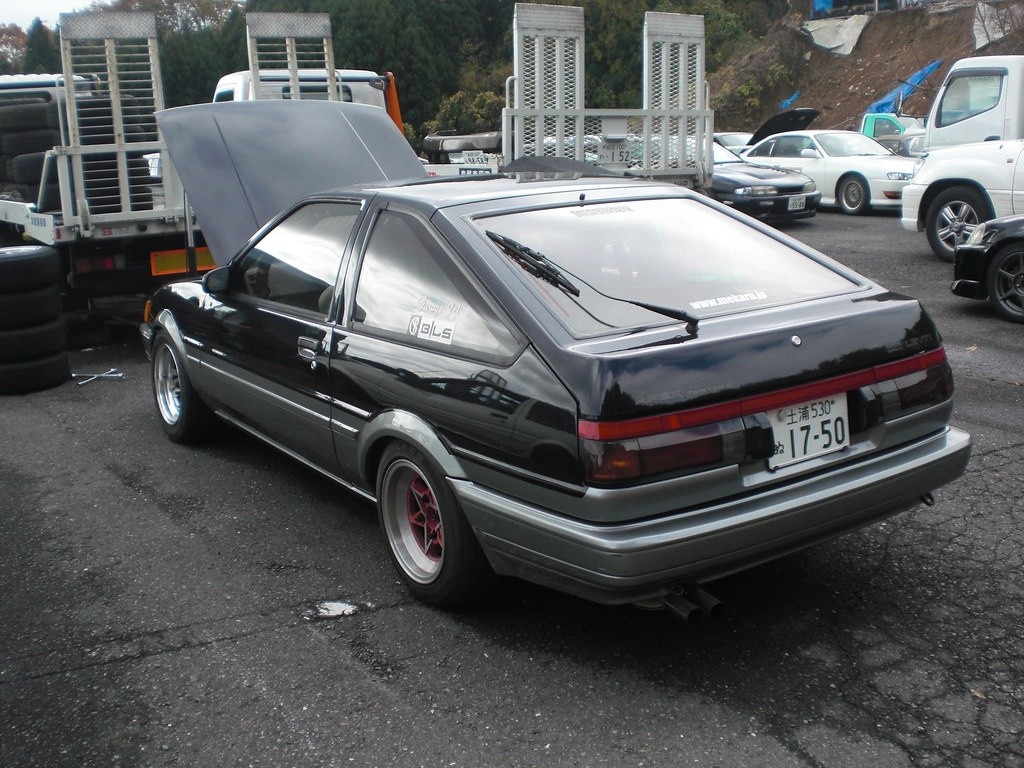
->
[947,212,1024,320]
[417,110,932,225]
[140,102,972,612]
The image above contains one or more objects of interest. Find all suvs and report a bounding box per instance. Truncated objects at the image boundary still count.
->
[901,137,1024,259]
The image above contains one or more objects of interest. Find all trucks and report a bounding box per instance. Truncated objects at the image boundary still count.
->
[0,9,342,320]
[924,53,1024,156]
[204,63,493,182]
